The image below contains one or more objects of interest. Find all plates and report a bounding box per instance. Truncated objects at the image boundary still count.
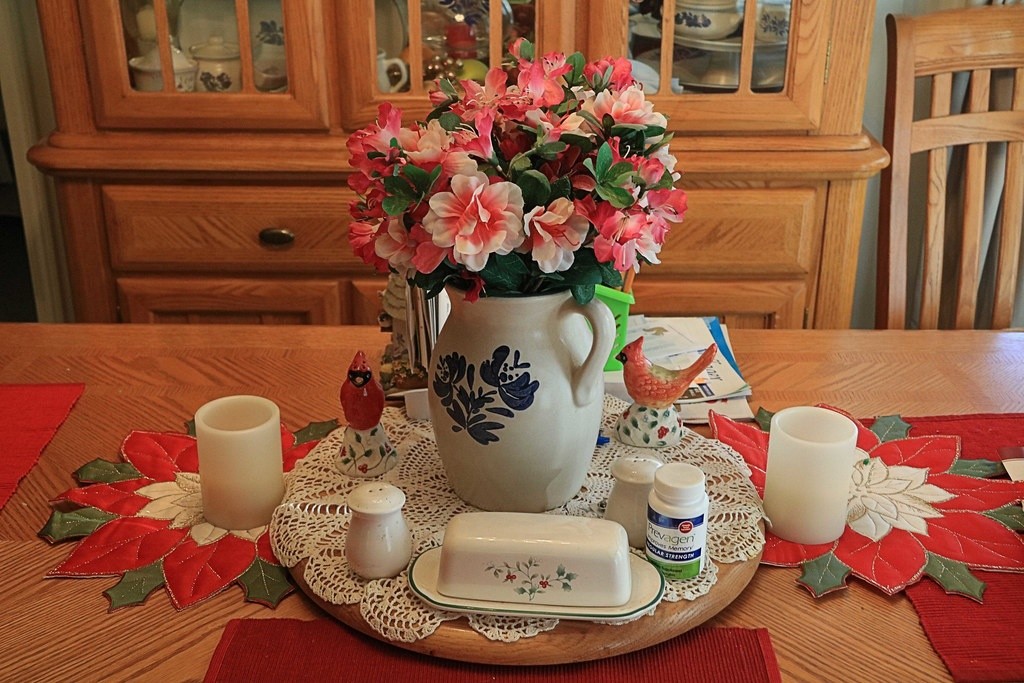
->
[405,545,667,624]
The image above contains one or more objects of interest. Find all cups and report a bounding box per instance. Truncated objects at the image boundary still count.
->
[376,47,408,94]
[764,405,858,545]
[195,395,284,530]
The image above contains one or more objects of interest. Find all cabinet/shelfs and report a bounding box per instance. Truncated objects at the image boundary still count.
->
[27,0,890,331]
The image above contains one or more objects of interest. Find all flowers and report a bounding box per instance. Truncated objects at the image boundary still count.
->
[347,36,690,304]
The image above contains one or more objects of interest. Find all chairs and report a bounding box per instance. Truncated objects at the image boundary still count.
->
[875,6,1023,331]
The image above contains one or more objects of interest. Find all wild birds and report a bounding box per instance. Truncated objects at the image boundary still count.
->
[614,335,718,409]
[338,350,385,430]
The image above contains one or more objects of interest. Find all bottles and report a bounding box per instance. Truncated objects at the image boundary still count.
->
[644,462,709,582]
[343,482,412,580]
[604,453,660,547]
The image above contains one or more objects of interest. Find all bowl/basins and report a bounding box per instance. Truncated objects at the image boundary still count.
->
[126,33,244,95]
[671,0,743,41]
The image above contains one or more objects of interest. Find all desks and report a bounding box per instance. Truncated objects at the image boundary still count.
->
[0,321,1024,683]
